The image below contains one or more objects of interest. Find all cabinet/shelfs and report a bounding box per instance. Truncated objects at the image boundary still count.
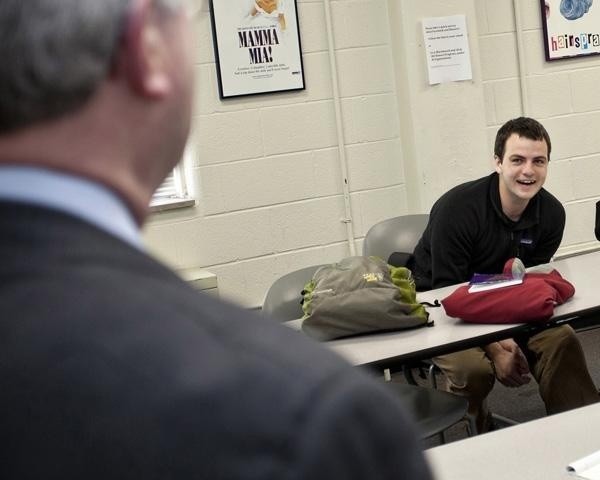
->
[169,265,221,310]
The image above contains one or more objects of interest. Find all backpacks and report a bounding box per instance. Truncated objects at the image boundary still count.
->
[301,256,429,340]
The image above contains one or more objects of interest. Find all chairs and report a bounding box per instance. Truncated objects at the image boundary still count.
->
[257,254,482,455]
[362,215,478,288]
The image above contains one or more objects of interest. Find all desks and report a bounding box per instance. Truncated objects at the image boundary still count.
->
[418,402,600,479]
[270,245,599,401]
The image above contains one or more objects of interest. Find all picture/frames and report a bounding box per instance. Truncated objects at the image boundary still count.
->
[209,0,311,101]
[541,0,600,62]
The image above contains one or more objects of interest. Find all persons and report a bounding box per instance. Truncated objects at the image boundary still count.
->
[403,117,600,446]
[1,0,434,479]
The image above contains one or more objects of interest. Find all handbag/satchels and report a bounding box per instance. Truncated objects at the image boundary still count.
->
[441,257,574,323]
